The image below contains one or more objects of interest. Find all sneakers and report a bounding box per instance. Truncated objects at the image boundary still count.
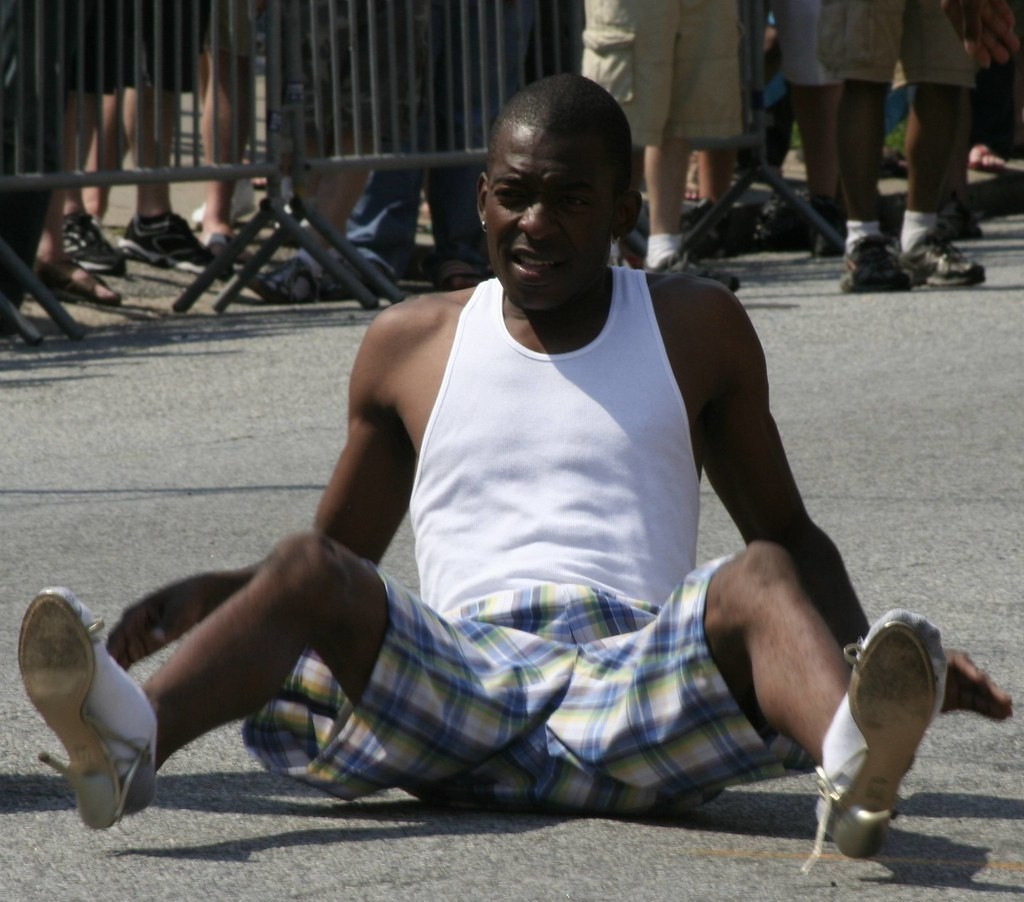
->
[118,213,215,274]
[60,211,126,273]
[839,233,913,293]
[893,231,986,286]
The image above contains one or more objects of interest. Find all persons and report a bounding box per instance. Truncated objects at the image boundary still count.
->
[16,70,1015,875]
[0,0,1024,335]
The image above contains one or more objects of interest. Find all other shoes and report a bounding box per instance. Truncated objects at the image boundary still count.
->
[434,260,478,290]
[32,260,122,308]
[341,246,404,306]
[188,182,257,231]
[812,234,841,256]
[247,256,318,303]
[203,232,254,263]
[640,254,740,294]
[681,153,699,199]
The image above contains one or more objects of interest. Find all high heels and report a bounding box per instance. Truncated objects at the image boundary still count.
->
[16,584,152,830]
[802,621,936,875]
